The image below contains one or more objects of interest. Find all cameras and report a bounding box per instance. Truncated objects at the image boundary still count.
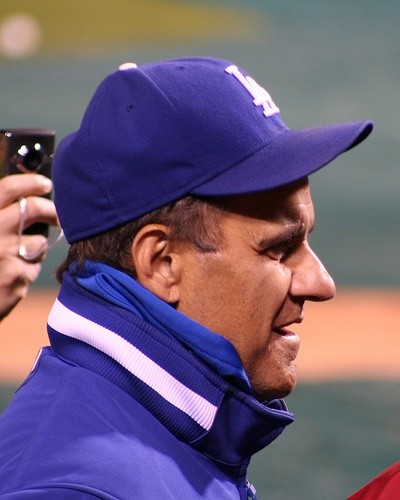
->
[0,129,56,263]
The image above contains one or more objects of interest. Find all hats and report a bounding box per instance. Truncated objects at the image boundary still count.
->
[50,56,374,245]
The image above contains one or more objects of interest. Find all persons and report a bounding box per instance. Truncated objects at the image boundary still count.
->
[1,172,63,320]
[2,56,374,499]
